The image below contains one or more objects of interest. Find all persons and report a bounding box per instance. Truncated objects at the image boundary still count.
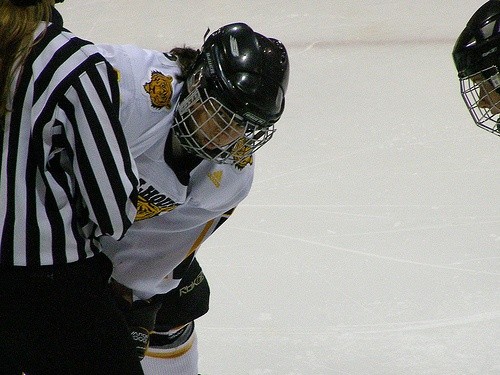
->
[451,0,500,140]
[96,22,290,374]
[0,0,144,375]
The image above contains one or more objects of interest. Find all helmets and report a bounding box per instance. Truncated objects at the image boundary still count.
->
[451,0,500,81]
[197,21,290,130]
